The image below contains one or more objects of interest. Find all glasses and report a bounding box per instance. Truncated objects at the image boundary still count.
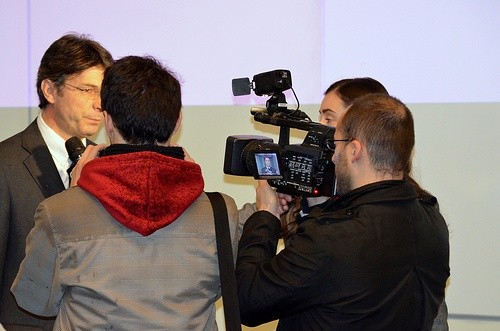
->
[326,139,352,152]
[62,81,102,100]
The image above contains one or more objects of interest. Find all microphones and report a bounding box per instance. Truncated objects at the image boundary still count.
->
[65,136,86,164]
[250,107,307,118]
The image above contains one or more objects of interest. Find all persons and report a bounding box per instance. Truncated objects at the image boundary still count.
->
[234,76,450,331]
[260,157,277,174]
[8,54,238,331]
[0,31,115,331]
